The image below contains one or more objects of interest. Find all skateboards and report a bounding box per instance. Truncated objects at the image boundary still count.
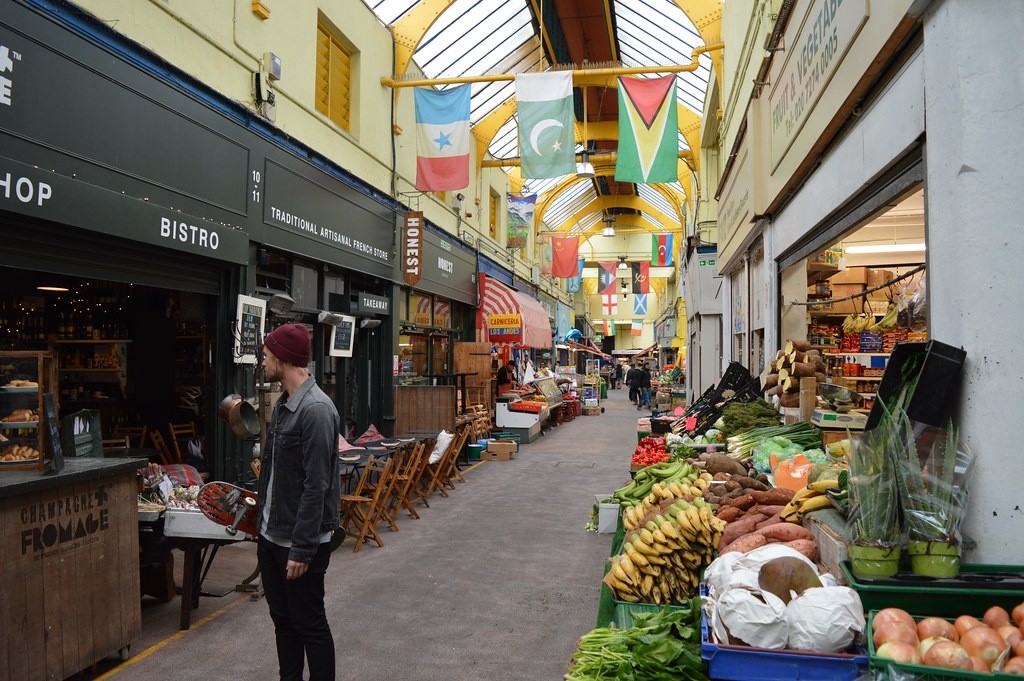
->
[195,481,348,553]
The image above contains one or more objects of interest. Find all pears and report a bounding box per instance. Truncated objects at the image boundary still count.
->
[757,556,823,603]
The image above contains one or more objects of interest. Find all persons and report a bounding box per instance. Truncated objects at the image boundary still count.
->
[495,360,522,397]
[254,323,347,681]
[609,363,625,390]
[625,362,654,412]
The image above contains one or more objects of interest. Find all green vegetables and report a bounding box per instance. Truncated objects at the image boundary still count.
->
[564,596,714,681]
[664,441,701,458]
[719,397,784,437]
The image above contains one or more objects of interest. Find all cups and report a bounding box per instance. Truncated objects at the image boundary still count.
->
[433,378,437,386]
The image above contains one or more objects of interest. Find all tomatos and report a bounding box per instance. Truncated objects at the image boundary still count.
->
[511,403,541,412]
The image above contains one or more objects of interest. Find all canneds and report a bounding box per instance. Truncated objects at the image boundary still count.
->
[831,366,843,377]
[93,330,100,340]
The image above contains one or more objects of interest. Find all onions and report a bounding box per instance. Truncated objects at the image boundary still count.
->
[872,599,1024,677]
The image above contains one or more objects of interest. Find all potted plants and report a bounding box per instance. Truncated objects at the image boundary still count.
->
[835,415,901,582]
[865,391,982,576]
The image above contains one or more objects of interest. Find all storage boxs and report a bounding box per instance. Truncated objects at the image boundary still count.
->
[808,266,893,314]
[594,494,619,534]
[583,399,600,416]
[651,341,1024,681]
[469,432,521,461]
[163,487,247,540]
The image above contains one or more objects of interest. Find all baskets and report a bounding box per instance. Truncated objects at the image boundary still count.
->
[611,597,689,632]
[670,361,765,440]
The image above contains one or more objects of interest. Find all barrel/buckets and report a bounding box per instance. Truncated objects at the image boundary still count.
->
[468,443,482,458]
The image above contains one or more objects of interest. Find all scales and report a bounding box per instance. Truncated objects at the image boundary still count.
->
[811,383,868,431]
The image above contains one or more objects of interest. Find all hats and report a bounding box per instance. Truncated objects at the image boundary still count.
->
[508,360,517,367]
[264,323,311,366]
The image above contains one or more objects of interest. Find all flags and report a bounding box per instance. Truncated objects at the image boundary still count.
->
[413,83,471,192]
[631,319,643,336]
[601,294,618,315]
[603,319,616,337]
[597,262,617,294]
[650,234,673,267]
[613,75,679,183]
[511,72,578,180]
[633,294,647,315]
[551,235,579,278]
[631,261,651,294]
[566,259,585,293]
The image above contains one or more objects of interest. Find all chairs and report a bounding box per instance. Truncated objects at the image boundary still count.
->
[101,423,472,550]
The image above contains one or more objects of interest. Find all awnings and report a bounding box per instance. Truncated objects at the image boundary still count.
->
[632,342,657,363]
[475,277,553,350]
[569,339,614,361]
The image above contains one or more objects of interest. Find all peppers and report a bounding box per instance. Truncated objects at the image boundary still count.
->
[631,435,671,464]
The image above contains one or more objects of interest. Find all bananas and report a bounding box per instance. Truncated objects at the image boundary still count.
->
[779,480,840,523]
[842,301,908,332]
[606,458,728,604]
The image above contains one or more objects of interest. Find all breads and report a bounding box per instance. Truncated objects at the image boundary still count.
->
[0,379,38,461]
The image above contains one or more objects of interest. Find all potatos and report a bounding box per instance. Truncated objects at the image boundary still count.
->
[625,455,819,564]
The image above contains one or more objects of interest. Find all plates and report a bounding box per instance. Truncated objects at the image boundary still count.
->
[0,456,39,462]
[0,386,38,392]
[0,421,41,422]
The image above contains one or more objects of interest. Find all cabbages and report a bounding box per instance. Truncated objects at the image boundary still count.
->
[666,417,727,445]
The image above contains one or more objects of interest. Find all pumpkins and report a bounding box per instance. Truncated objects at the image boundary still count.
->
[768,453,849,493]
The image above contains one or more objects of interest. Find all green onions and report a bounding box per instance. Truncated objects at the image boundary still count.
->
[727,420,823,460]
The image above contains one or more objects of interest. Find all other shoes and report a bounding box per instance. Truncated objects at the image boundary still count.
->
[637,406,641,409]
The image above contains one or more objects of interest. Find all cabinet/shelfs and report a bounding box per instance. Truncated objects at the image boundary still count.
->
[0,455,148,681]
[812,324,936,410]
[0,339,133,472]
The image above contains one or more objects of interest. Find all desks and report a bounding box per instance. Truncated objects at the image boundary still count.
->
[331,430,441,515]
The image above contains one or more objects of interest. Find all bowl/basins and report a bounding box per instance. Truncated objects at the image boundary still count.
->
[138,511,159,521]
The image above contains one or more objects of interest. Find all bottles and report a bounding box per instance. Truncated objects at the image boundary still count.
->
[399,362,403,372]
[56,297,125,340]
[823,355,845,377]
[181,322,187,336]
[0,297,44,350]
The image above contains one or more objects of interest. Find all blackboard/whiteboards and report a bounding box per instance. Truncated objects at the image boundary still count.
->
[42,393,65,471]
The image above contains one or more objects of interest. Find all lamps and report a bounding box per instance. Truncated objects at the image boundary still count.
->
[575,151,629,302]
